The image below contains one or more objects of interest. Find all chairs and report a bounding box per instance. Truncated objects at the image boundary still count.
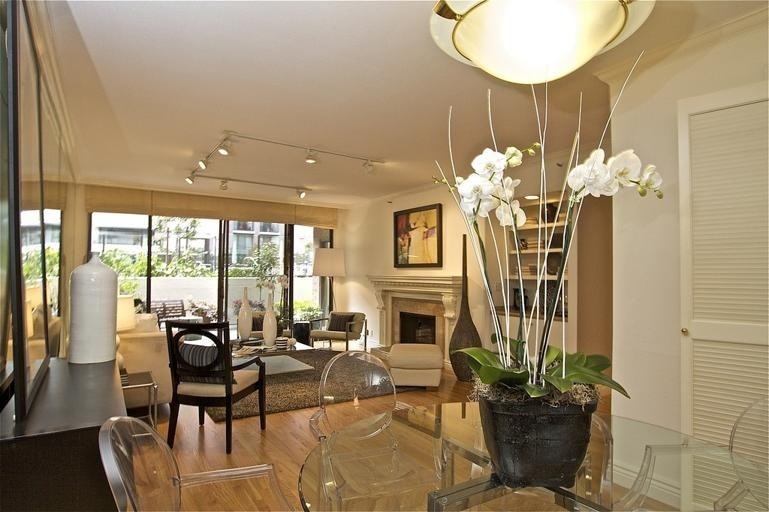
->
[120,323,265,454]
[712,402,766,512]
[310,309,368,350]
[305,350,422,512]
[94,414,297,512]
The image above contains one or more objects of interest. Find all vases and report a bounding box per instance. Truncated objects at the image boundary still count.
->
[67,253,115,364]
[237,286,277,346]
[475,393,598,490]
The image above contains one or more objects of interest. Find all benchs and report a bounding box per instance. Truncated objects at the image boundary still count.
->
[149,300,186,329]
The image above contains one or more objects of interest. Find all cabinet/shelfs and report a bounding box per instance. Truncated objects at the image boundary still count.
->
[0,360,128,512]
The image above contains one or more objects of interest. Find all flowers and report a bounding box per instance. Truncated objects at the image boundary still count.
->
[411,49,664,401]
[232,274,292,327]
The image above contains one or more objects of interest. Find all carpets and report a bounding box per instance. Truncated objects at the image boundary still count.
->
[203,348,423,422]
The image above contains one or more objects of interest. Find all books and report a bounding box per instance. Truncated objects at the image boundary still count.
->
[515,264,541,275]
[527,240,548,250]
[526,217,538,224]
[275,337,289,349]
[236,345,272,355]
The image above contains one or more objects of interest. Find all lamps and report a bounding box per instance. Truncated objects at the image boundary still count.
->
[312,247,346,312]
[116,296,138,370]
[296,150,317,199]
[428,1,657,85]
[184,143,230,184]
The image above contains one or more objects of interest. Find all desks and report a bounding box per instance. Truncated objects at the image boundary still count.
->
[299,404,767,511]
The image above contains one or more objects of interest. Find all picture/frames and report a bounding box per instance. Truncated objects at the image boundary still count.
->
[394,204,442,268]
[7,5,47,425]
